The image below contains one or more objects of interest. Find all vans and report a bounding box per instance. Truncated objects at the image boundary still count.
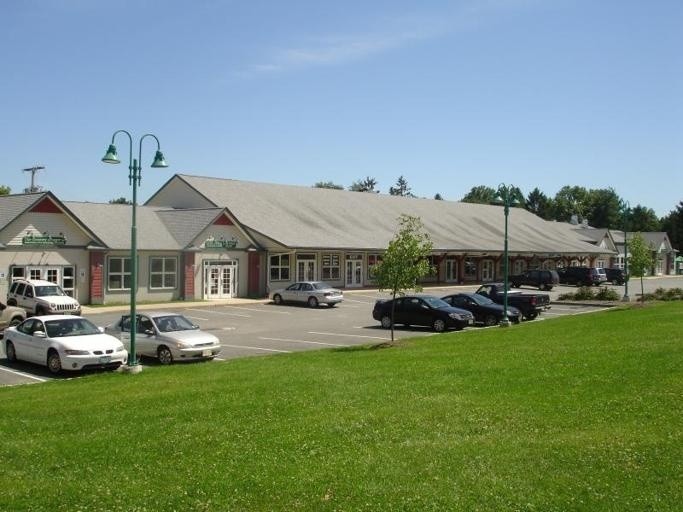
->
[556,266,607,287]
[507,268,559,291]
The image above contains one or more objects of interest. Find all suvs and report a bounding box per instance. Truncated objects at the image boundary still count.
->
[6,279,80,318]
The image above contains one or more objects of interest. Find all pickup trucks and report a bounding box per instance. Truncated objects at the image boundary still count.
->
[474,282,551,320]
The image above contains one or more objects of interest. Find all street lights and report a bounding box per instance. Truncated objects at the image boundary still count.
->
[491,183,520,328]
[100,130,168,375]
[616,198,633,302]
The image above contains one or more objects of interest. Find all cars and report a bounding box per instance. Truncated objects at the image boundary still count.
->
[0,301,26,335]
[104,311,222,365]
[595,268,629,286]
[372,294,475,332]
[268,281,343,308]
[2,314,127,375]
[430,293,520,327]
